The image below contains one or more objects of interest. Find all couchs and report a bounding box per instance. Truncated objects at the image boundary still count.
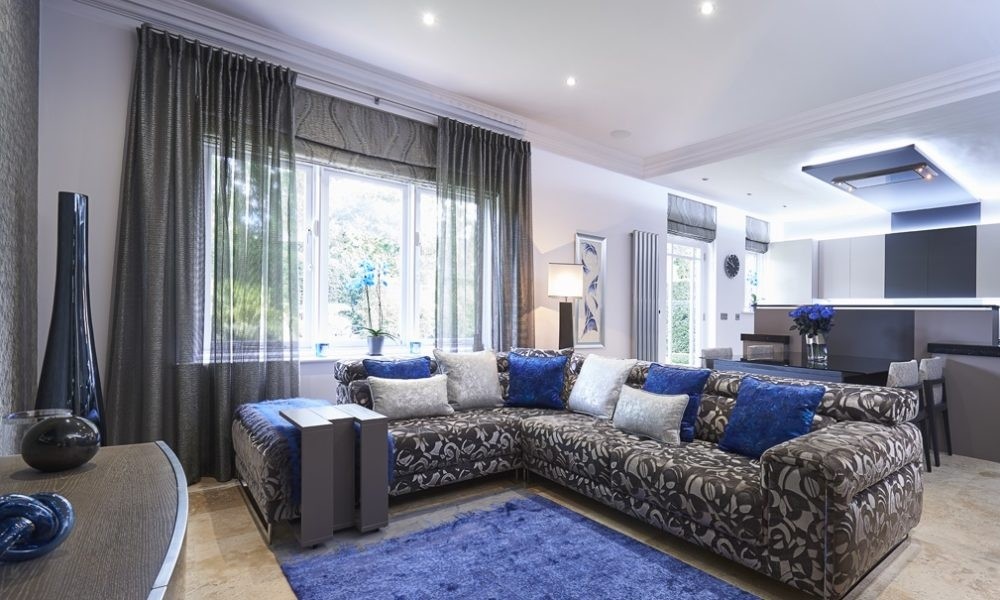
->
[334,348,924,600]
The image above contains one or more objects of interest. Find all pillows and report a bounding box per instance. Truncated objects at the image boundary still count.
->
[643,362,713,443]
[716,376,824,458]
[367,375,454,419]
[504,351,568,409]
[613,384,690,446]
[566,353,637,419]
[433,348,504,409]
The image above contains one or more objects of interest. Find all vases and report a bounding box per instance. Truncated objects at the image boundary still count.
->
[805,333,828,360]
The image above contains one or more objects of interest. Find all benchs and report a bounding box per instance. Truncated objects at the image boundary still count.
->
[231,397,394,549]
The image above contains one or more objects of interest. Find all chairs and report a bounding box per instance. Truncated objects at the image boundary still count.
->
[892,382,931,472]
[923,375,952,466]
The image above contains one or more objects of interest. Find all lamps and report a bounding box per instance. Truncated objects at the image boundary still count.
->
[548,263,584,350]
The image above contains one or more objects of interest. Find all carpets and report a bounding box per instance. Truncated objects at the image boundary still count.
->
[274,490,762,600]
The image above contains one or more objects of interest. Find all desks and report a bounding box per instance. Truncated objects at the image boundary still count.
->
[0,441,188,600]
[280,402,389,545]
[698,351,904,378]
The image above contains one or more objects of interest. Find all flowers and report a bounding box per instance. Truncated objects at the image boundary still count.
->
[789,305,836,337]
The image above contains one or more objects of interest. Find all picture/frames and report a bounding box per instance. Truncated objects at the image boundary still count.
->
[574,231,605,349]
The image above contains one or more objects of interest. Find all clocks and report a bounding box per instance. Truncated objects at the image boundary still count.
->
[726,254,740,278]
